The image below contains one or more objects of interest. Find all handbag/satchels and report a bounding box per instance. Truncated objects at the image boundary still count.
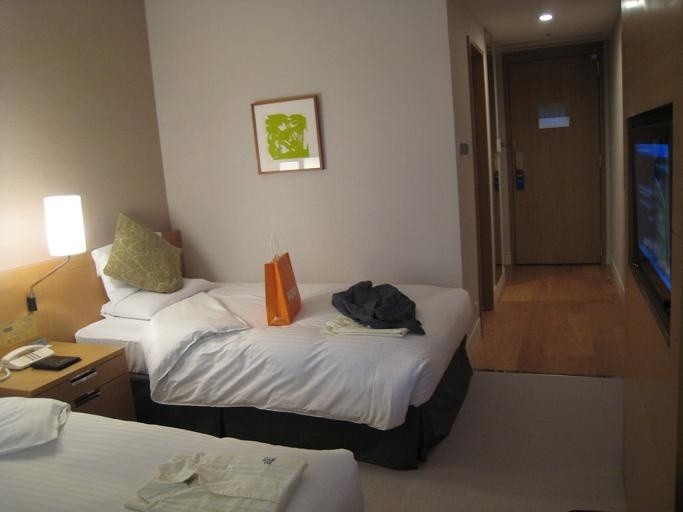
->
[262,233,301,326]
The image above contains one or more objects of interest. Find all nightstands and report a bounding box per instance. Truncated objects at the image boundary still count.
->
[0,341,137,421]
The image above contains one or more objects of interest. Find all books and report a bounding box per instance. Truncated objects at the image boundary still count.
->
[31,355,82,371]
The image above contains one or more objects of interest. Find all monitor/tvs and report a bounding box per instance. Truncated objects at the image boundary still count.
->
[630,121,675,306]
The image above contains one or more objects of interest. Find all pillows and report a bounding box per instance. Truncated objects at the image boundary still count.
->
[91,231,162,307]
[100,278,216,322]
[104,212,183,293]
[0,397,69,456]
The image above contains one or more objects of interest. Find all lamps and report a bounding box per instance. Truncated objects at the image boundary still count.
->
[27,195,87,313]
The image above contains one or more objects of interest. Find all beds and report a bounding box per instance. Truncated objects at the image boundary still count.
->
[0,395,362,512]
[75,280,472,470]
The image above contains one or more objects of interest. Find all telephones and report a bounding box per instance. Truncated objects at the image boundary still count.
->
[1,344,55,371]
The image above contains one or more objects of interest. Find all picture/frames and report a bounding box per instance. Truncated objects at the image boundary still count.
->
[250,96,324,174]
